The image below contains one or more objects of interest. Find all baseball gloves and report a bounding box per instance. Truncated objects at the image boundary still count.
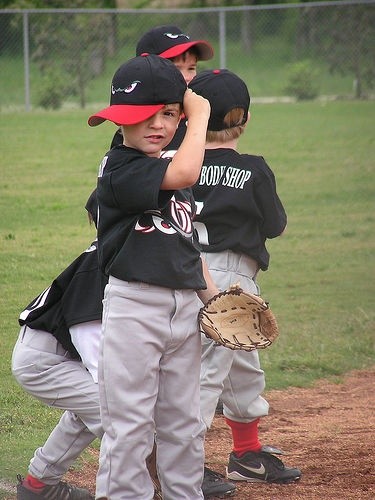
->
[198,281,278,352]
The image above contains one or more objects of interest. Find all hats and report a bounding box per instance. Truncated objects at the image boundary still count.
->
[88,53,187,127]
[136,26,214,61]
[187,69,250,131]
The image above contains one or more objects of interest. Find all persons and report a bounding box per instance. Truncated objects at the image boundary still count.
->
[9,236,108,500]
[84,52,221,500]
[187,67,304,499]
[110,25,215,160]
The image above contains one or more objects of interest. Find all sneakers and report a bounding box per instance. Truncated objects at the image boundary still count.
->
[226,446,301,483]
[16,474,93,500]
[201,468,236,496]
